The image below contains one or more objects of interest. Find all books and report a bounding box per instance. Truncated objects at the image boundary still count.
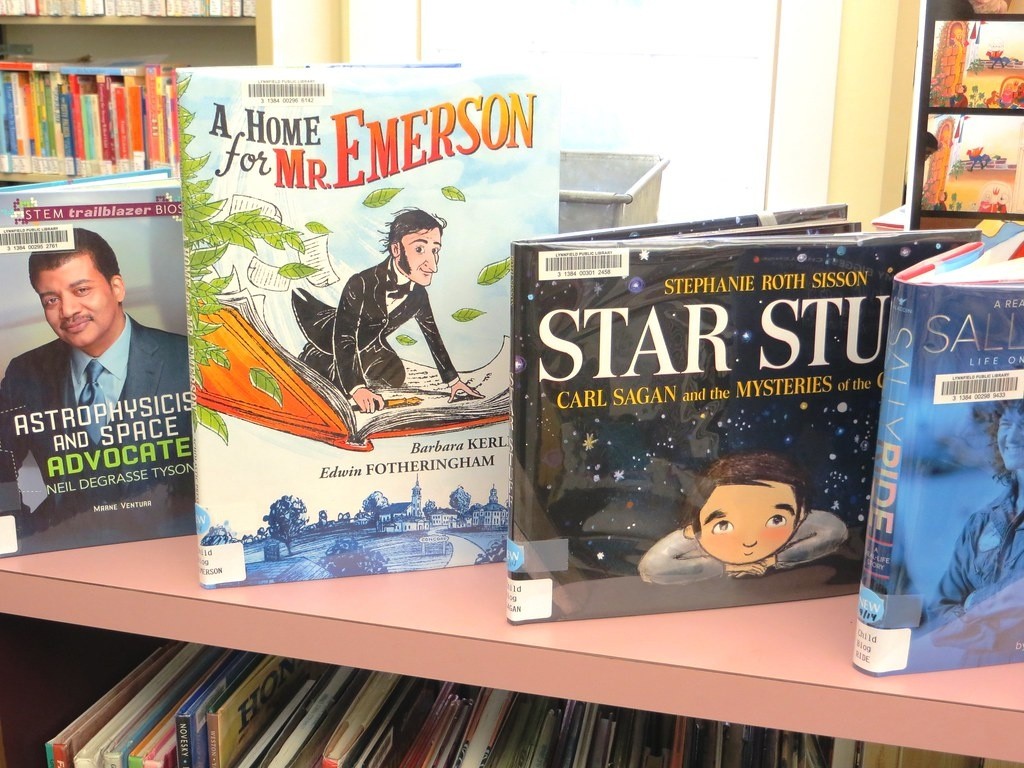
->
[172,63,558,589]
[43,639,1024,768]
[852,238,1024,680]
[0,169,197,559]
[505,204,982,625]
[0,56,191,178]
[0,0,256,18]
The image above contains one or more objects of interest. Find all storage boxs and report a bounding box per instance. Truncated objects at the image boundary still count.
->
[559,153,669,234]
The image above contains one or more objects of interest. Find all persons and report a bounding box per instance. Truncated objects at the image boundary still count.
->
[0,229,195,557]
[932,363,1024,625]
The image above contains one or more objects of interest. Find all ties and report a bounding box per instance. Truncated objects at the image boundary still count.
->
[77,359,108,445]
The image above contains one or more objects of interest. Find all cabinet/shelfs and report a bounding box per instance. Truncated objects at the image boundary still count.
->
[905,0,1024,230]
[0,0,341,182]
